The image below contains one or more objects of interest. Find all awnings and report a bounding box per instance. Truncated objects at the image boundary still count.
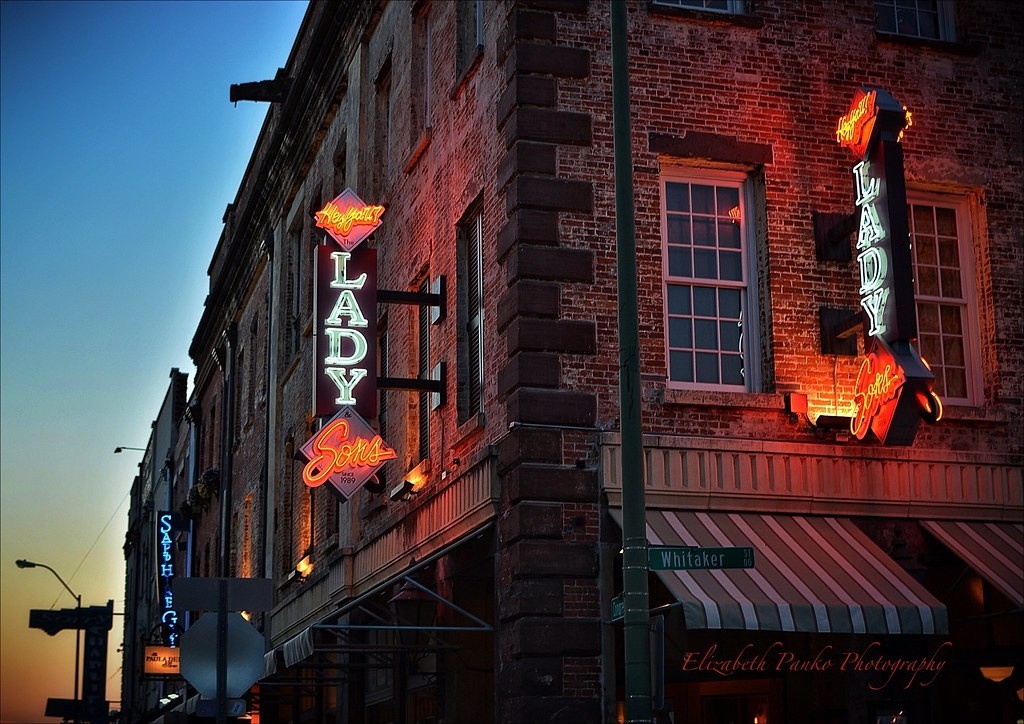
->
[152,522,496,724]
[921,519,1024,609]
[610,507,948,635]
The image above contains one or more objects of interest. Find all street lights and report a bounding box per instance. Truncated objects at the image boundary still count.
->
[15,558,82,702]
